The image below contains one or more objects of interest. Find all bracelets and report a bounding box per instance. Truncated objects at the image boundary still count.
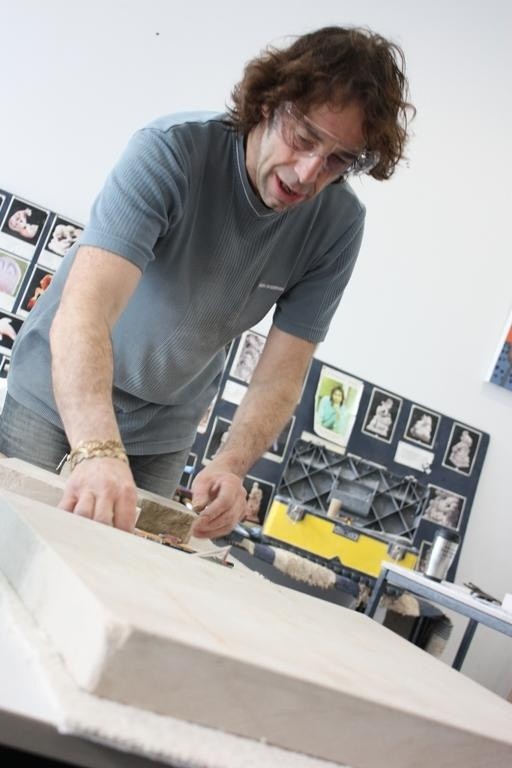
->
[66,440,129,470]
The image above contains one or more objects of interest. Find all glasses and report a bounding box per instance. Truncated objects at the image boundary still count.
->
[276,100,378,177]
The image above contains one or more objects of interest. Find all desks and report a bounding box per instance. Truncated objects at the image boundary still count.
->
[362,560,512,672]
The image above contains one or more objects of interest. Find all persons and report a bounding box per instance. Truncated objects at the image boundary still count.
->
[320,385,347,434]
[0,26,417,541]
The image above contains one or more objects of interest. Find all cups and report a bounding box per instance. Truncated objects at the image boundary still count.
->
[326,498,342,519]
[423,528,460,583]
